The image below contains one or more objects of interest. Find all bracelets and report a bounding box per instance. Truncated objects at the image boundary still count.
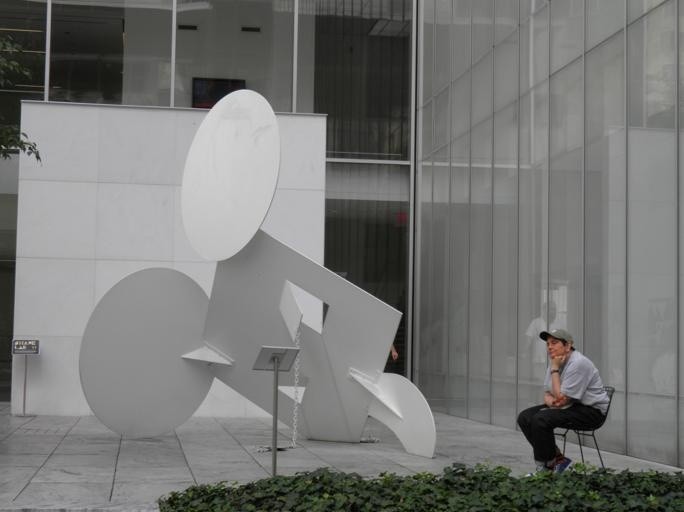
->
[551,370,559,374]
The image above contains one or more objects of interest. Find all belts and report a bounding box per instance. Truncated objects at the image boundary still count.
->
[591,409,601,414]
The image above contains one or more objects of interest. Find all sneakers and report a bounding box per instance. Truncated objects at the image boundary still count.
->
[524,456,574,477]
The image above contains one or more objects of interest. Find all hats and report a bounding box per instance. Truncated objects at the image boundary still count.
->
[539,328,576,351]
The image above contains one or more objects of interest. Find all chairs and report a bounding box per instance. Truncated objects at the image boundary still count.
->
[554,386,615,476]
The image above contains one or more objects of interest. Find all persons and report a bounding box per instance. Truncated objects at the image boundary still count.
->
[520,301,568,406]
[517,329,610,477]
[391,345,398,360]
